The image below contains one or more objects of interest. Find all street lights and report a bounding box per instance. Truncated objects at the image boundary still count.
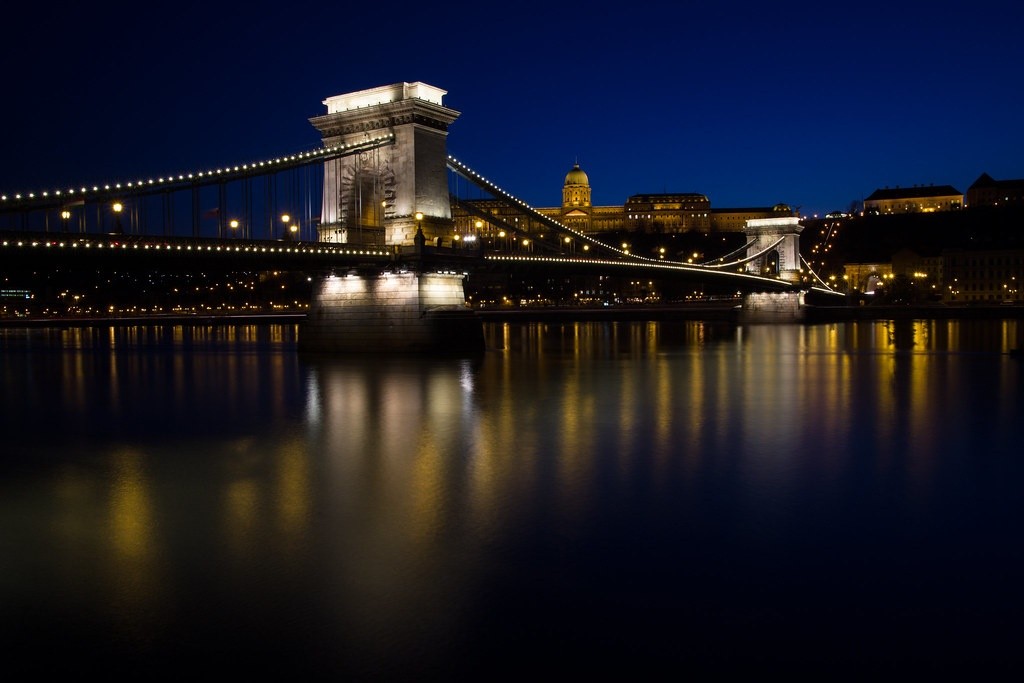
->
[414,213,426,245]
[621,243,629,263]
[230,219,238,237]
[282,214,290,241]
[660,248,664,265]
[565,237,574,255]
[291,225,296,240]
[476,221,483,249]
[584,245,589,258]
[112,202,123,235]
[693,252,698,267]
[499,232,505,252]
[453,235,460,248]
[62,210,70,231]
